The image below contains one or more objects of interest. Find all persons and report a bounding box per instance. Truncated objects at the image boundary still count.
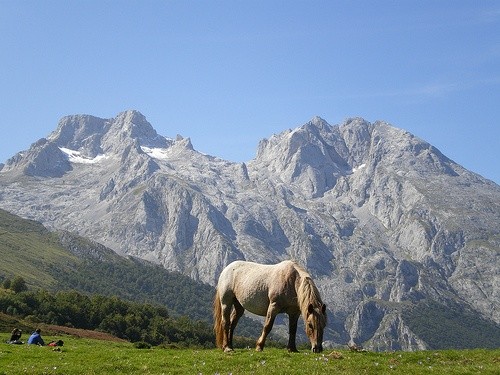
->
[9,327,22,342]
[27,328,46,347]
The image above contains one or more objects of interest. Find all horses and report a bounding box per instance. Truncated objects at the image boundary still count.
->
[213,260,327,352]
